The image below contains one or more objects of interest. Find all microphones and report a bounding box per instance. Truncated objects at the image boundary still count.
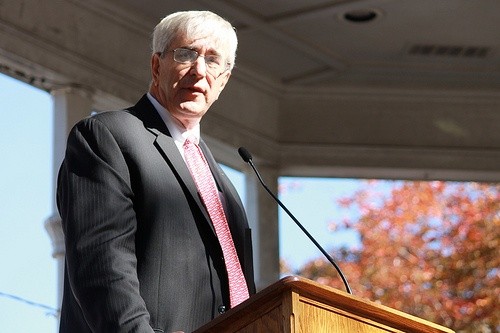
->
[238,147,352,295]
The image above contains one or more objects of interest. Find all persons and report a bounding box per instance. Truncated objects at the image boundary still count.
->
[57,12,257,333]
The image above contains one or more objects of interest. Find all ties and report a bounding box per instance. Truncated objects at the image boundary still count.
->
[182,139,250,308]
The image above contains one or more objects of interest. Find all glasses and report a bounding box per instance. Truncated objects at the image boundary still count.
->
[158,48,230,75]
